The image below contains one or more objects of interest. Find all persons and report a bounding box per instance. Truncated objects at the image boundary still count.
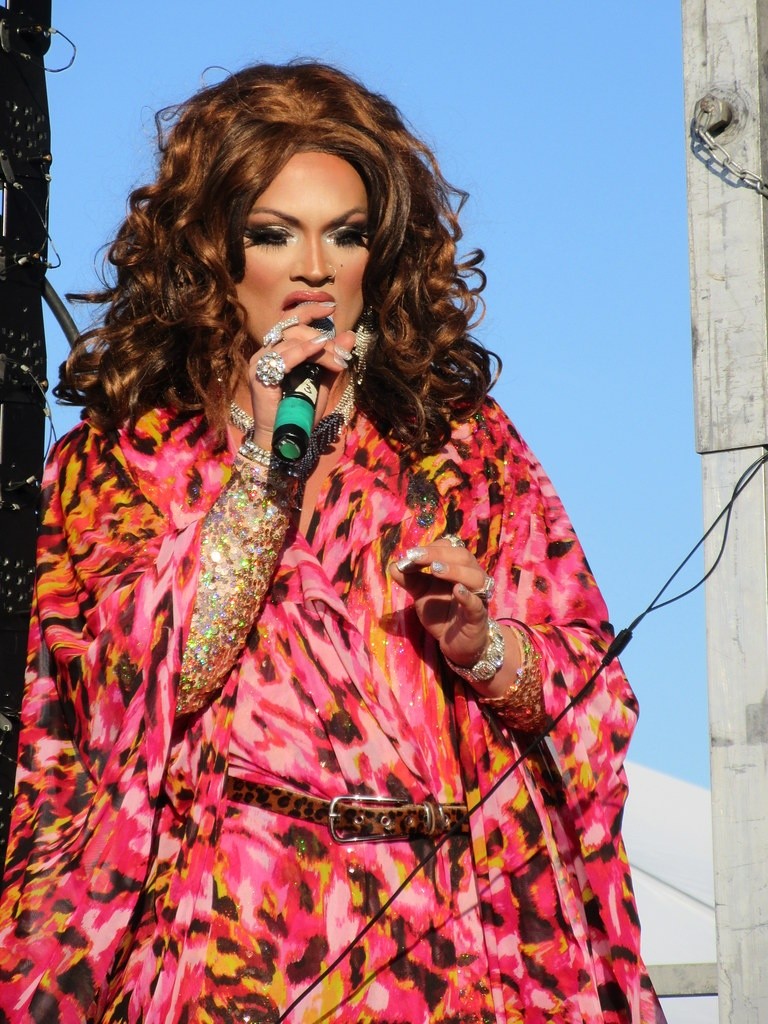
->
[0,59,668,1024]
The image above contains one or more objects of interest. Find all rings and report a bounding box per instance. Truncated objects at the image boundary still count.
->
[261,317,298,346]
[444,534,465,548]
[474,577,496,601]
[255,352,285,386]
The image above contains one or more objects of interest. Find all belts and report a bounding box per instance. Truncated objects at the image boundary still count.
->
[221,775,472,843]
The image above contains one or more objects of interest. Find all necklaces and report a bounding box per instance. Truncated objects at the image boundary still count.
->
[227,381,357,469]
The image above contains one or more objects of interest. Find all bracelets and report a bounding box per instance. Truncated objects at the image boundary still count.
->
[477,626,547,734]
[445,618,503,683]
[239,441,308,477]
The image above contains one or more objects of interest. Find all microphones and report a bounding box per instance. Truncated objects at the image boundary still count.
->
[272,314,337,461]
